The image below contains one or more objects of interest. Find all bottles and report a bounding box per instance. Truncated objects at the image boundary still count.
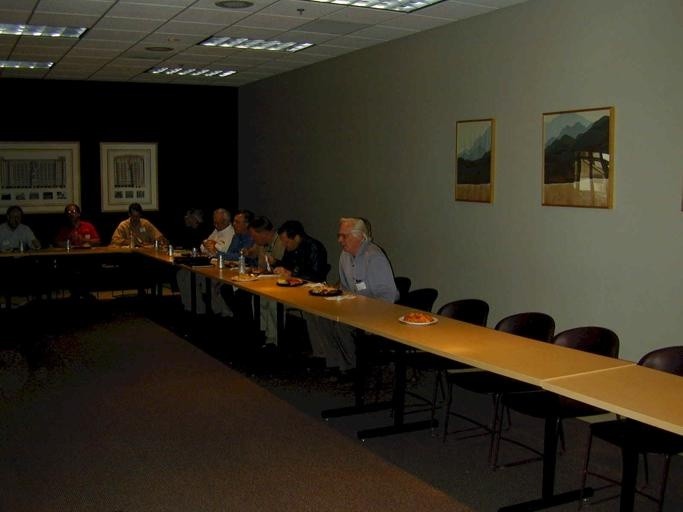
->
[263,254,272,271]
[128,230,136,250]
[237,248,247,274]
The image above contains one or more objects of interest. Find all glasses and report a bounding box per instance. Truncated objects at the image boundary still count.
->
[337,231,354,239]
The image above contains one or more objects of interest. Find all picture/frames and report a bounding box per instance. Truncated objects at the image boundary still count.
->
[99,141,159,213]
[539,106,616,208]
[453,119,497,204]
[0,140,82,215]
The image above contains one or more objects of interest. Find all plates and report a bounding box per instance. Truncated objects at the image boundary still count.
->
[275,279,308,288]
[250,271,282,278]
[397,312,439,327]
[308,288,343,297]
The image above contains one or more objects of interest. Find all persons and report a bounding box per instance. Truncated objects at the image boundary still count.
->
[247,221,328,354]
[174,208,234,323]
[218,214,284,328]
[0,205,43,254]
[204,211,253,320]
[111,203,167,302]
[306,218,399,388]
[55,204,100,303]
